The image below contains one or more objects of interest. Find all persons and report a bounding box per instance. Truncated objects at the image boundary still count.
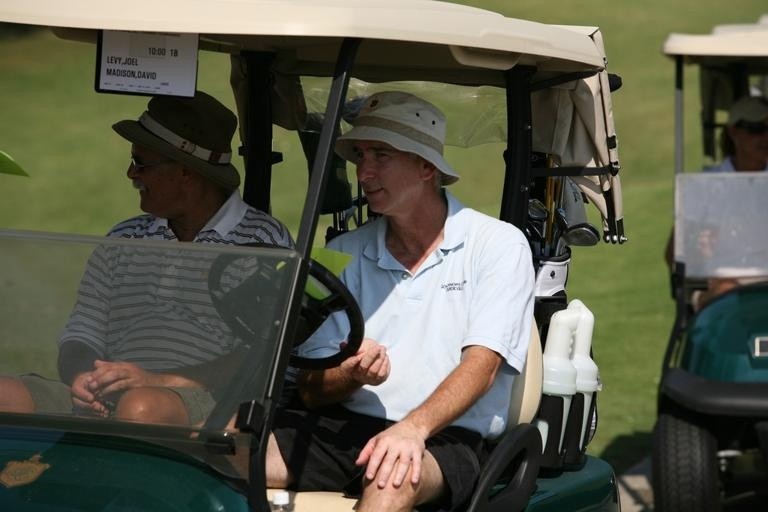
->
[191,91,535,512]
[0,90,296,426]
[664,97,768,314]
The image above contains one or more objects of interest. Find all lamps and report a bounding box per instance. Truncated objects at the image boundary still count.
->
[264,314,546,512]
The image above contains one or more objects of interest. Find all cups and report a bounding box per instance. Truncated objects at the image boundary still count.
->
[130,150,176,172]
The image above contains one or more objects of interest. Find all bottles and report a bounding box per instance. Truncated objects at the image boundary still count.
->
[729,96,768,127]
[112,91,240,190]
[332,91,459,185]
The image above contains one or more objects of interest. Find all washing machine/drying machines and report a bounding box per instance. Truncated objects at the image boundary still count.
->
[302,96,368,233]
[523,152,600,256]
